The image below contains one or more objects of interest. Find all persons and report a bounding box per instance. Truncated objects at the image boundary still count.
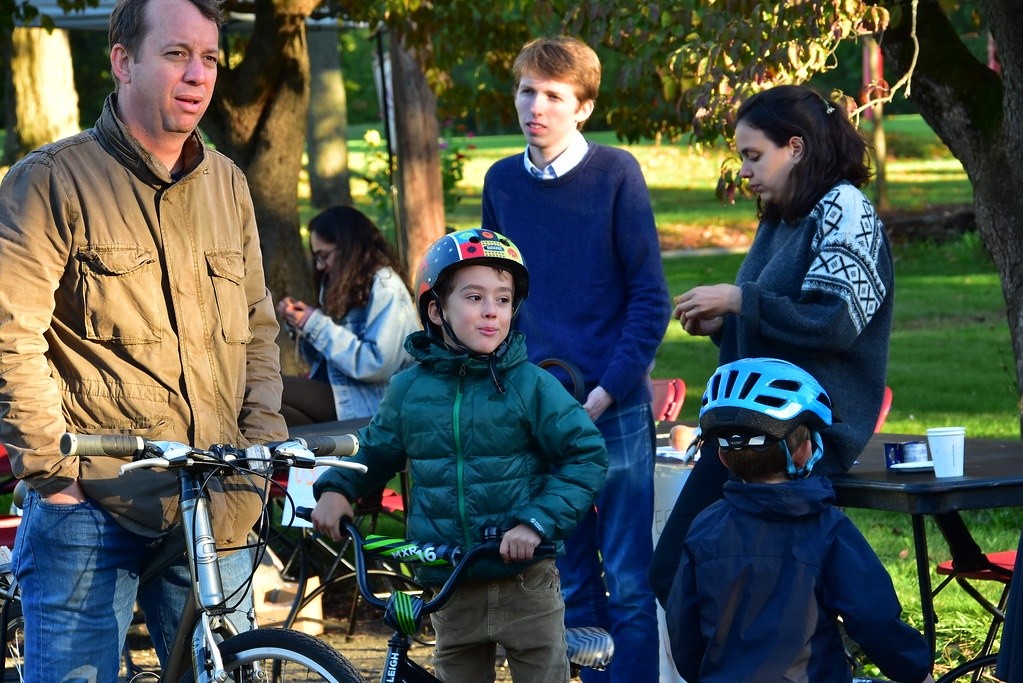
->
[482,35,670,683]
[312,229,609,683]
[1,0,289,683]
[665,357,936,683]
[278,205,423,429]
[650,84,894,611]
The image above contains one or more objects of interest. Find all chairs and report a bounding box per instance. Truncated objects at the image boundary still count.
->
[931,509,1021,683]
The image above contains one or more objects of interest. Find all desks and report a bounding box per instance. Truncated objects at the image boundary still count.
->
[642,434,1023,677]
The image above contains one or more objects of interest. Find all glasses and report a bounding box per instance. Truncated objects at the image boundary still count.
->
[312,246,339,268]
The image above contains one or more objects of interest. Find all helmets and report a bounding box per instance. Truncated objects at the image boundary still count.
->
[414,229,529,338]
[698,358,834,438]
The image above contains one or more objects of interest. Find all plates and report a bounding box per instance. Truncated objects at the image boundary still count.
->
[890,461,934,472]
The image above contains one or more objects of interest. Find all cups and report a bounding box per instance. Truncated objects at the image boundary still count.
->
[927,426,965,478]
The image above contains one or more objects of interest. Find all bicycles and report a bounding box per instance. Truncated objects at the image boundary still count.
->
[0,432,558,683]
